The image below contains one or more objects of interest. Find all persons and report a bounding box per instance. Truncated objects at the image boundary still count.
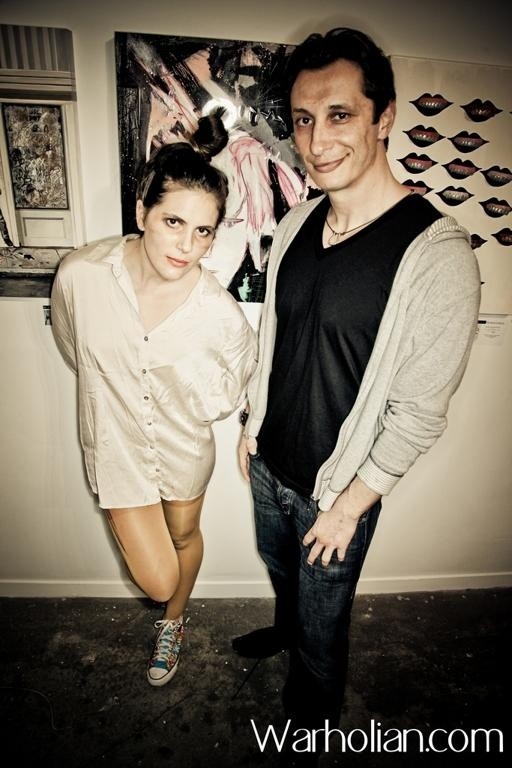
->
[232,27,482,768]
[51,106,259,688]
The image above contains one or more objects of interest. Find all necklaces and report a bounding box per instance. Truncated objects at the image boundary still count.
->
[325,217,378,246]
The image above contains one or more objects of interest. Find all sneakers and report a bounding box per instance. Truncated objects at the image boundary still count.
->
[231,626,285,658]
[148,616,183,686]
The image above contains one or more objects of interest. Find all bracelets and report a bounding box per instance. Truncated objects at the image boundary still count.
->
[239,413,248,426]
